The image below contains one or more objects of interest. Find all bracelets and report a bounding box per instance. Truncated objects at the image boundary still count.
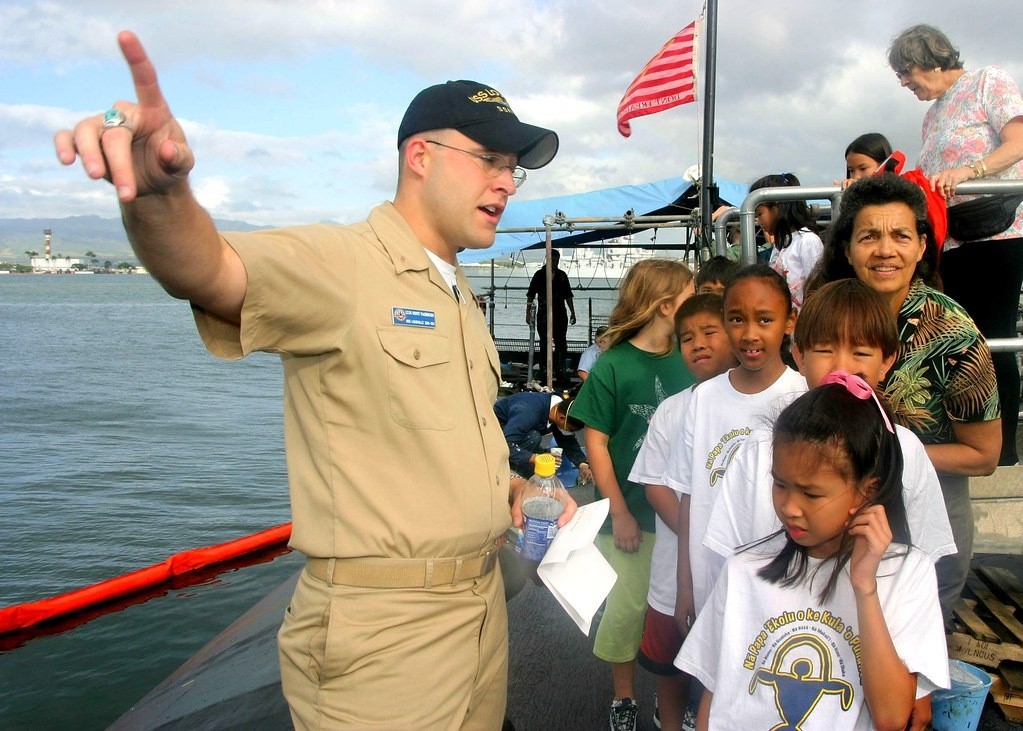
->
[977,159,987,177]
[510,473,525,481]
[965,164,980,180]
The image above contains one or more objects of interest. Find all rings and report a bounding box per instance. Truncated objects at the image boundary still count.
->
[97,109,135,140]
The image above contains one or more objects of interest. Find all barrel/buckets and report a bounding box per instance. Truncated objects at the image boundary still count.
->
[926,659,992,731]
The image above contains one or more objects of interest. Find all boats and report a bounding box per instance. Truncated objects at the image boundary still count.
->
[74,271,94,275]
[523,235,657,279]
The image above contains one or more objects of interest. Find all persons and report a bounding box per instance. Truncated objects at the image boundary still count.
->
[889,24,1023,466]
[54,30,560,731]
[493,132,1023,731]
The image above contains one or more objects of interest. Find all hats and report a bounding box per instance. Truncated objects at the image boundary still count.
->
[596,326,610,336]
[397,80,559,170]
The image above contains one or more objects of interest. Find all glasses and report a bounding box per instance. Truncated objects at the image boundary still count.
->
[895,61,917,79]
[426,140,526,188]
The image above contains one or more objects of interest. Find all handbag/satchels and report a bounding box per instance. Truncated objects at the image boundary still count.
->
[946,193,1023,242]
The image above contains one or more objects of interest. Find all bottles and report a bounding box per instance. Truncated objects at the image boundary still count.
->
[518,455,563,562]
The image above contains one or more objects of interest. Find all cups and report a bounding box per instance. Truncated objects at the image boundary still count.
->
[549,447,564,468]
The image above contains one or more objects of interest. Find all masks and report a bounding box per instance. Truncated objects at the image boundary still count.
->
[557,427,573,436]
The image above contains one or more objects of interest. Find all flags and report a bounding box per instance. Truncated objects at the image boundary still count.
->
[617,19,706,138]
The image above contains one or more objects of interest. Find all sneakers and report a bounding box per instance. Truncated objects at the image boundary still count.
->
[608,696,640,731]
[653,692,697,731]
